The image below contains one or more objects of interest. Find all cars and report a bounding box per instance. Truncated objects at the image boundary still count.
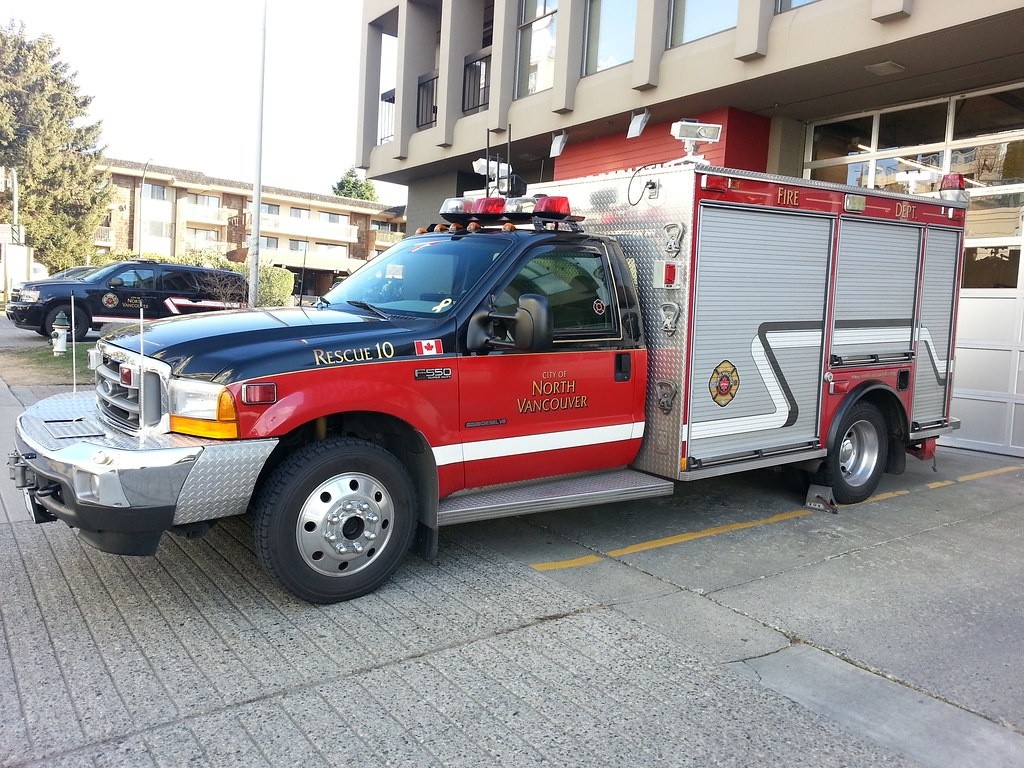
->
[47,265,97,279]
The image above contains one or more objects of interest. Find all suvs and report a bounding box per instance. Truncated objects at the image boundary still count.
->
[6,258,252,341]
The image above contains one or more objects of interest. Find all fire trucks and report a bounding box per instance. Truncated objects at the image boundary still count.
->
[5,119,974,609]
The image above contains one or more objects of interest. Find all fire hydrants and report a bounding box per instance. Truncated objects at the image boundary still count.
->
[49,310,71,356]
